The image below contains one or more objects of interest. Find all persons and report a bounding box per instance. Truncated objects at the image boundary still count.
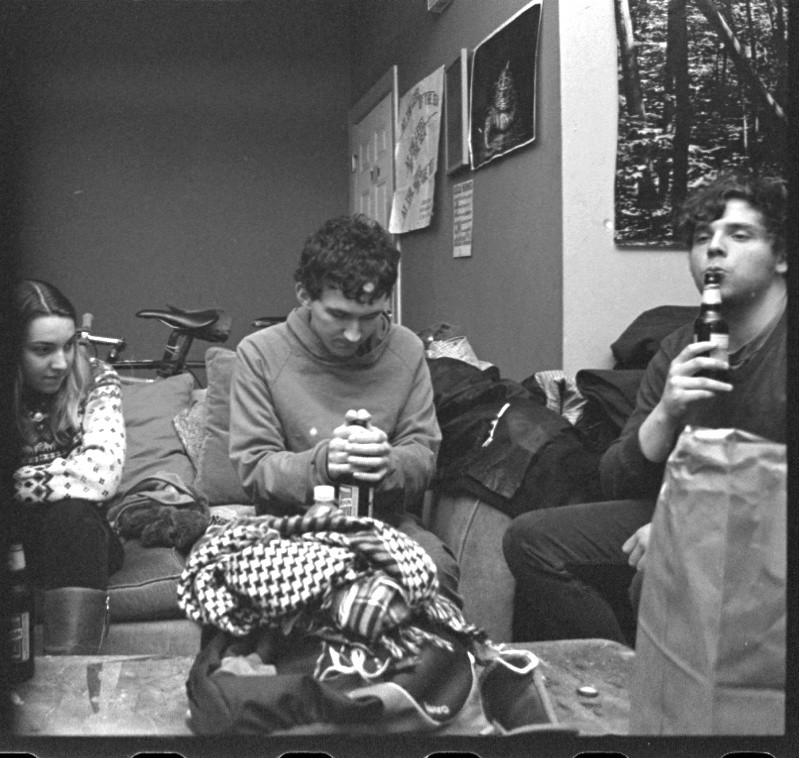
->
[0,279,126,657]
[229,216,463,612]
[503,183,790,646]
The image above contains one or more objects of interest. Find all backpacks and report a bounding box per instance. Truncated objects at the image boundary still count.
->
[184,603,580,741]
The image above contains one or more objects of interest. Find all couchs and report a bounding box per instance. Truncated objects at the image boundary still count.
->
[33,347,643,657]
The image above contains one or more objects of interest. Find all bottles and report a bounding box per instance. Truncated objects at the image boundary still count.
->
[337,419,374,517]
[303,485,340,519]
[693,273,729,395]
[0,538,35,682]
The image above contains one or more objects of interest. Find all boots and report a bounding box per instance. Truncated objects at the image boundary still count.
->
[42,586,111,657]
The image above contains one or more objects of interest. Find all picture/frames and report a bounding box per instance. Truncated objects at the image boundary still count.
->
[444,48,469,176]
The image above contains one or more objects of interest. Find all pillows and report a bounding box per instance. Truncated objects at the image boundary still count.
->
[193,347,253,505]
[118,373,193,493]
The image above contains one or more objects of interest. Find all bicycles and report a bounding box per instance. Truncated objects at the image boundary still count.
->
[74,309,289,392]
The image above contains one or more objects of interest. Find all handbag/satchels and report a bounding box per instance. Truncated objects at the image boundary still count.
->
[632,427,784,737]
